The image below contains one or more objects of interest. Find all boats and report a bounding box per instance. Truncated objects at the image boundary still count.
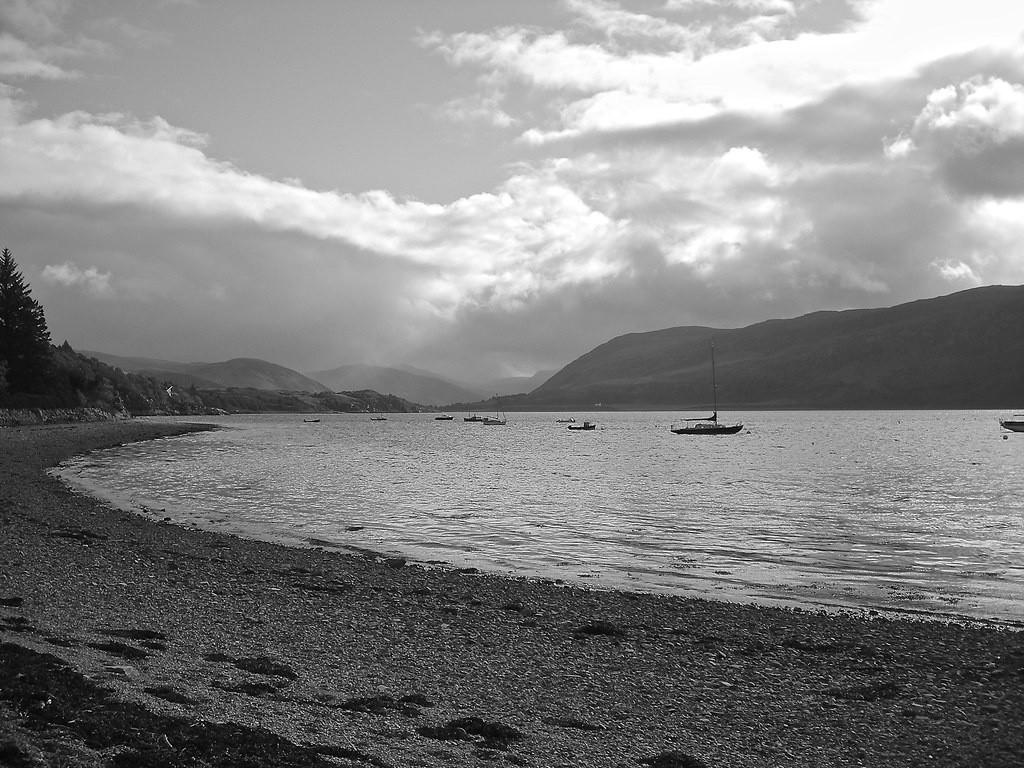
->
[371,415,387,421]
[998,414,1024,432]
[567,422,596,431]
[556,417,575,422]
[463,401,507,426]
[435,413,455,420]
[303,419,320,422]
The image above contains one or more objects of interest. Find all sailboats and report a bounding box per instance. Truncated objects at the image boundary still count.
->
[669,336,744,435]
